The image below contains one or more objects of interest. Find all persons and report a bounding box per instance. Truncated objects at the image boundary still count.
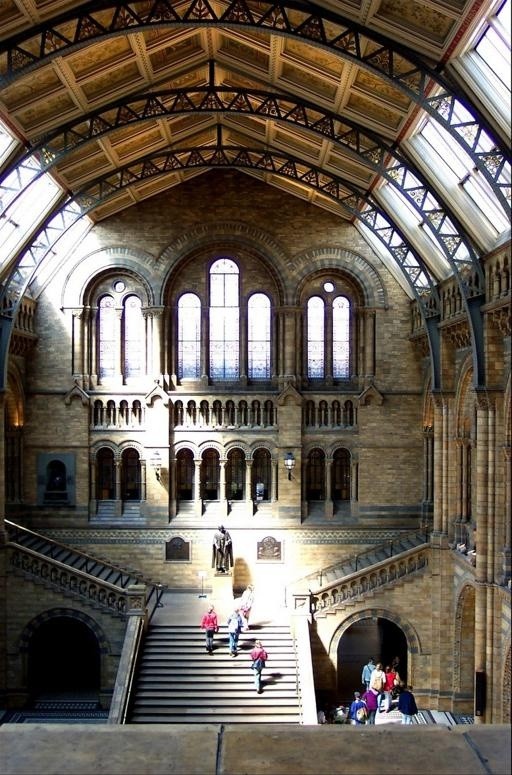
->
[227,608,244,657]
[239,584,255,631]
[250,640,268,694]
[317,655,418,725]
[212,525,233,572]
[466,528,476,556]
[201,604,219,655]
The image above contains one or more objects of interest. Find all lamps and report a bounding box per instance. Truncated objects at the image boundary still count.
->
[284,451,296,480]
